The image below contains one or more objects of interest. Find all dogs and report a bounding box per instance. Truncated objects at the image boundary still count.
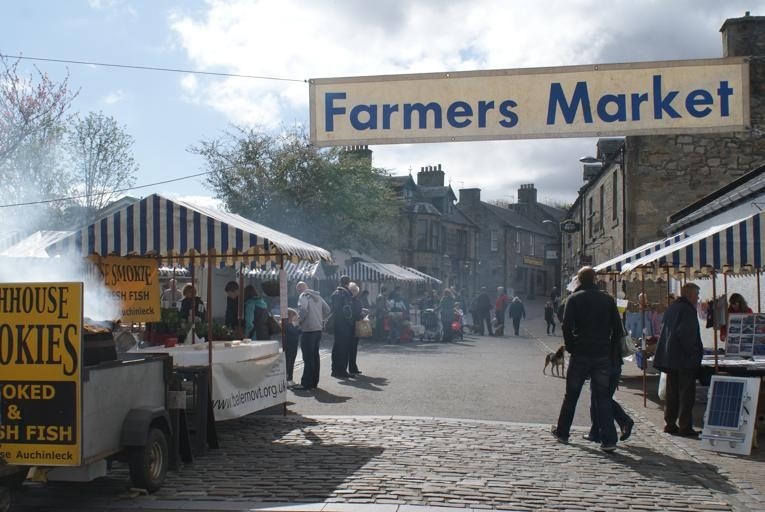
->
[543,345,566,378]
[463,323,481,335]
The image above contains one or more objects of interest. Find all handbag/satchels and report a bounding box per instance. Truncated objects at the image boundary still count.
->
[354,319,372,338]
[266,316,282,337]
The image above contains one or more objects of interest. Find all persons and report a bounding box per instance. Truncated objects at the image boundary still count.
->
[650,282,700,438]
[551,266,620,449]
[584,290,636,440]
[720,292,754,341]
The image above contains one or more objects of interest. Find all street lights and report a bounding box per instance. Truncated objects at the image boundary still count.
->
[463,255,481,297]
[542,220,564,302]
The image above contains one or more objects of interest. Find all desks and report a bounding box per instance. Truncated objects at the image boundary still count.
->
[701,357,765,448]
[176,350,287,450]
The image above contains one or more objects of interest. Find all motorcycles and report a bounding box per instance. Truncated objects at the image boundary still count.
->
[549,280,561,300]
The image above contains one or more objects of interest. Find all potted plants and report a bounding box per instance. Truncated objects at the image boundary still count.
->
[161,306,179,347]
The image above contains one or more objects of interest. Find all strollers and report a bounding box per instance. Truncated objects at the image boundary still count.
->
[420,309,442,342]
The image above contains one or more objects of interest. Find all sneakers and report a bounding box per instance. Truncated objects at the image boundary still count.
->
[286,380,320,391]
[620,416,634,441]
[550,424,568,444]
[330,369,361,378]
[583,433,601,442]
[664,425,703,437]
[599,442,616,450]
